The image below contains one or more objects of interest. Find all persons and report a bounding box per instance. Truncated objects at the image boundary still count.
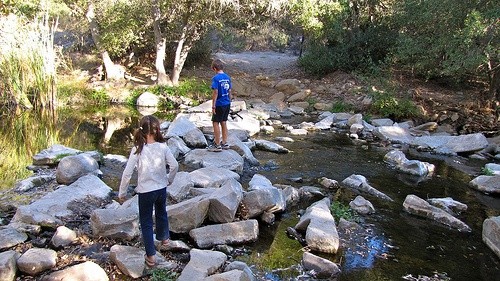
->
[206,59,232,152]
[118,116,179,266]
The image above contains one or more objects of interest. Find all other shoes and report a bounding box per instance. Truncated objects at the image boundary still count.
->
[162,237,171,246]
[220,141,229,149]
[143,252,156,267]
[206,143,222,151]
